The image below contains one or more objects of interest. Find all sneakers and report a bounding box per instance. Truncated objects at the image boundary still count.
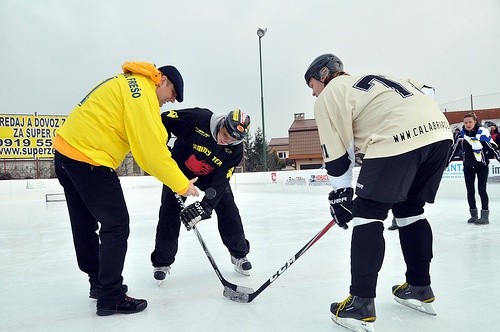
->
[230,256,252,277]
[89,283,128,299]
[330,295,376,332]
[152,265,171,286]
[95,292,148,316]
[392,282,437,316]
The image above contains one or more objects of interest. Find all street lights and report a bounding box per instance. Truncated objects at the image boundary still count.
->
[256,27,270,171]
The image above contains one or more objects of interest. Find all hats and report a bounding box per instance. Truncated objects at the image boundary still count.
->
[158,64,184,103]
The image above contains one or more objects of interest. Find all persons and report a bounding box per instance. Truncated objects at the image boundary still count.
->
[387,113,500,229]
[151,107,253,286]
[52,61,200,317]
[305,54,452,332]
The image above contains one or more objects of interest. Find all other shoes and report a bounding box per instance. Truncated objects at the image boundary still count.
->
[387,217,398,230]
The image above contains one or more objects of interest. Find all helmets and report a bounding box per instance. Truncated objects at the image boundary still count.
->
[224,109,251,141]
[304,53,343,85]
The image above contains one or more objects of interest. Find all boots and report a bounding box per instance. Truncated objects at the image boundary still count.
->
[466,208,478,223]
[474,209,490,225]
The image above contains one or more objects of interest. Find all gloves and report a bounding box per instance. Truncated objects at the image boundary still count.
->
[328,187,356,230]
[179,200,212,231]
